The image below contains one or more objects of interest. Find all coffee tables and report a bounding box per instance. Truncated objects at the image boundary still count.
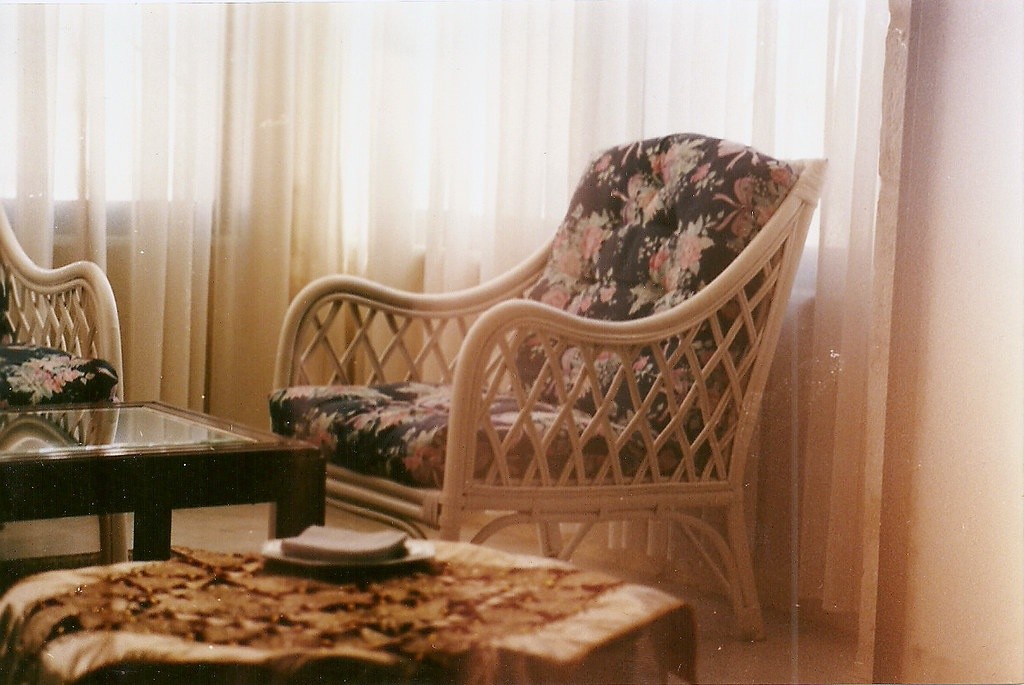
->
[0,399,330,565]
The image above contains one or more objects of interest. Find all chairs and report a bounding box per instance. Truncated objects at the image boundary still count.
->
[266,132,832,645]
[0,203,132,570]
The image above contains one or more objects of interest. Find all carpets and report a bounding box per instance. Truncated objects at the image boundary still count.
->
[0,548,133,599]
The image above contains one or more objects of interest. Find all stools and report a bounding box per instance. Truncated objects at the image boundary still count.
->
[1,527,701,685]
